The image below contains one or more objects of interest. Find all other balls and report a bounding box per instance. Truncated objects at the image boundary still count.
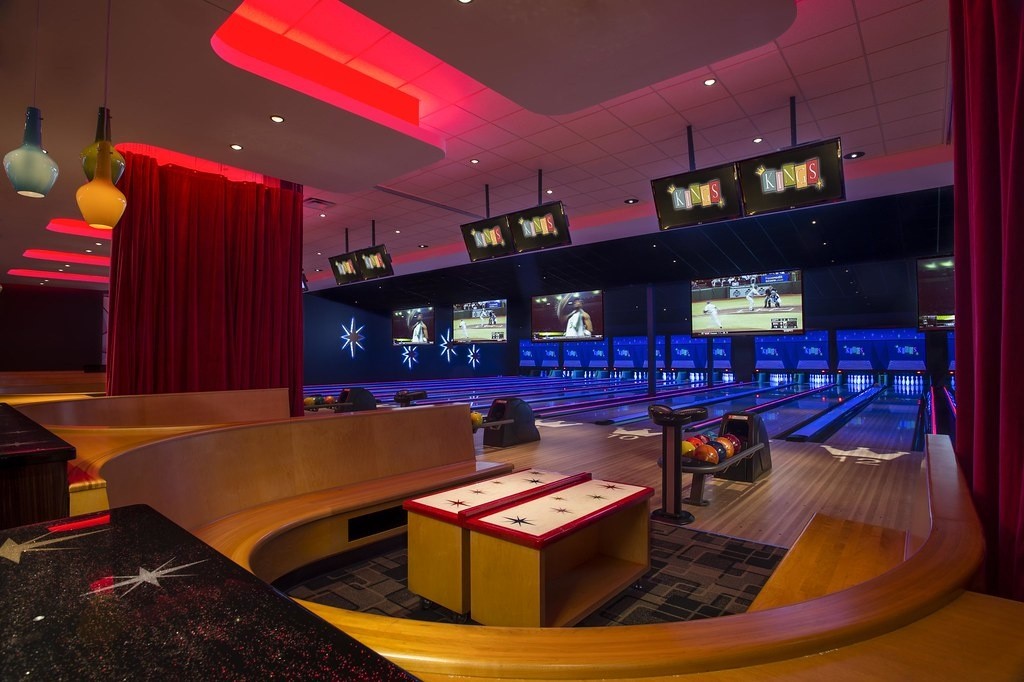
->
[681,429,746,465]
[471,411,484,426]
[304,395,335,405]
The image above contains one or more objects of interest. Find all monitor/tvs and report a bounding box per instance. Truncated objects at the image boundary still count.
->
[452,298,509,344]
[689,267,805,339]
[530,289,604,343]
[916,253,955,331]
[391,305,436,346]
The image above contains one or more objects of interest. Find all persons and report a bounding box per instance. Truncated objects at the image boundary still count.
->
[764,286,780,308]
[488,311,496,325]
[460,319,469,338]
[480,310,487,326]
[558,293,592,337]
[464,302,485,310]
[703,301,723,328]
[746,286,762,312]
[711,276,756,287]
[406,309,428,342]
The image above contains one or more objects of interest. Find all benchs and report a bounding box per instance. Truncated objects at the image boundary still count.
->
[743,510,911,613]
[187,460,515,575]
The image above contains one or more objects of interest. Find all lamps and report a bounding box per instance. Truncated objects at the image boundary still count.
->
[2,0,60,199]
[76,0,128,230]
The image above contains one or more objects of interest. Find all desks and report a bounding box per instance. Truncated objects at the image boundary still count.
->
[403,467,592,616]
[461,476,656,629]
[1,401,77,533]
[1,504,423,682]
